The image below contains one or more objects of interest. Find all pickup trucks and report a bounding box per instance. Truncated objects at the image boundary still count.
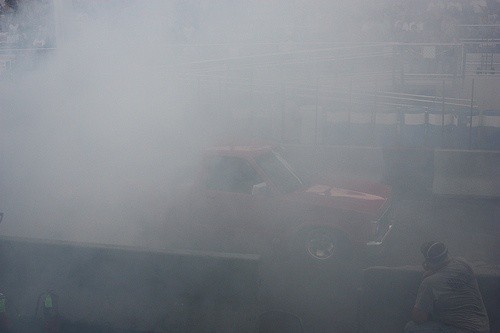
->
[122,138,394,262]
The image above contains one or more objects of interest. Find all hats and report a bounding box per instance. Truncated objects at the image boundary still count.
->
[423,241,448,261]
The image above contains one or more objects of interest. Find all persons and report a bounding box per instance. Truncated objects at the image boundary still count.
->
[402,241,490,333]
